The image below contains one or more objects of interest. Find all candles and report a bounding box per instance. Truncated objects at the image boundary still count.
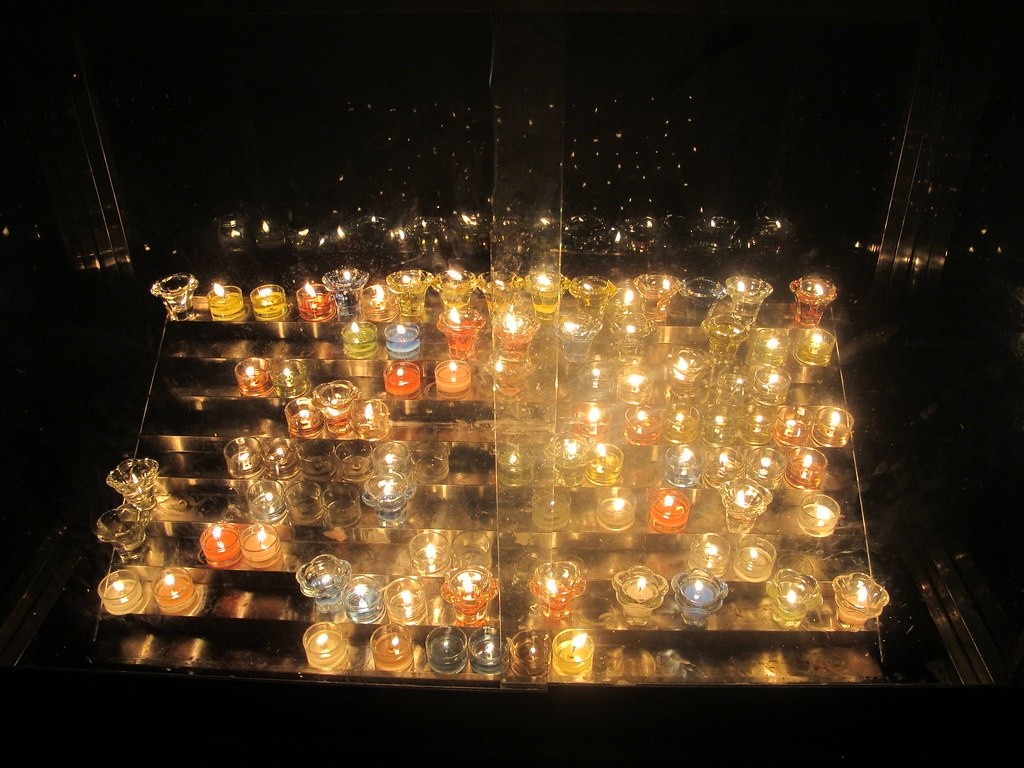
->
[93,198,948,678]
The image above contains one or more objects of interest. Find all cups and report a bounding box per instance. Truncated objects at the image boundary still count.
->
[94,210,891,678]
